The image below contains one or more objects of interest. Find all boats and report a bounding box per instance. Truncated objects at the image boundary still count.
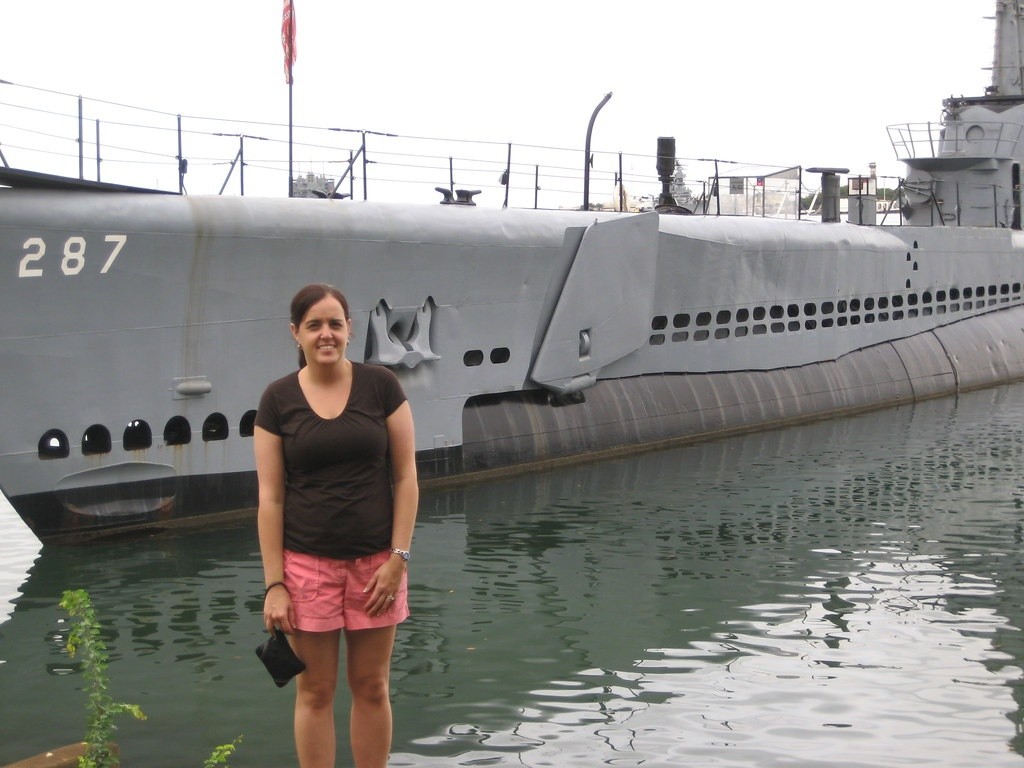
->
[0,0,1023,545]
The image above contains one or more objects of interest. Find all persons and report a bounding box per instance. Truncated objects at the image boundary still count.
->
[254,283,421,765]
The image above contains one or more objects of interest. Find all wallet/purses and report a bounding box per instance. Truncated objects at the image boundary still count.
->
[255,630,306,690]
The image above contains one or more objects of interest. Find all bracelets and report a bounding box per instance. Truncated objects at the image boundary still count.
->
[262,580,286,595]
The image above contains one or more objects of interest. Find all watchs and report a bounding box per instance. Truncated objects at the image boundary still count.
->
[390,547,412,562]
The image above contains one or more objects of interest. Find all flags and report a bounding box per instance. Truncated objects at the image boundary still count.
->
[280,0,298,85]
